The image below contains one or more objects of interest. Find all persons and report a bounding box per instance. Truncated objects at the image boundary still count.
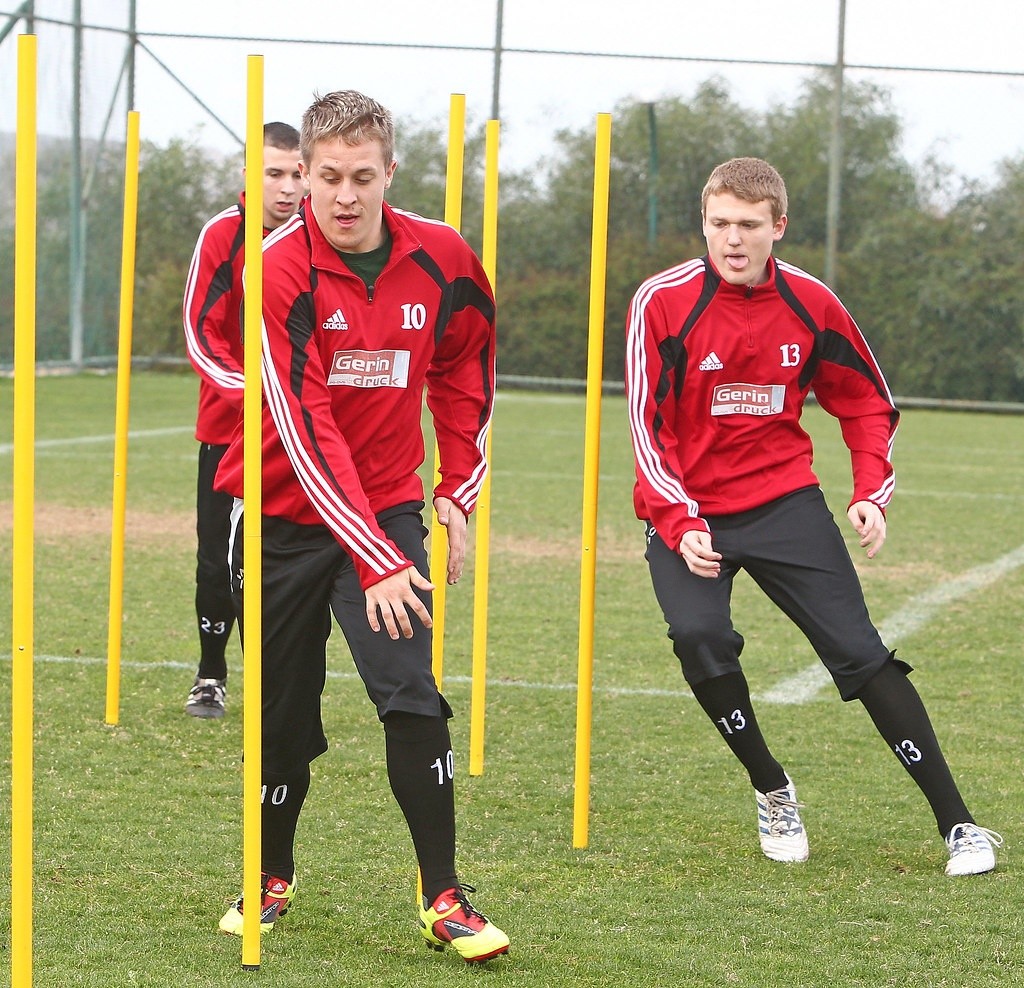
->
[623,157,1004,876]
[185,121,304,718]
[221,92,513,963]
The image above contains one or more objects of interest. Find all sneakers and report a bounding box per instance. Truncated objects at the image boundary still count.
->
[419,883,511,967]
[945,822,1003,876]
[186,674,227,719]
[219,868,298,937]
[755,771,809,863]
